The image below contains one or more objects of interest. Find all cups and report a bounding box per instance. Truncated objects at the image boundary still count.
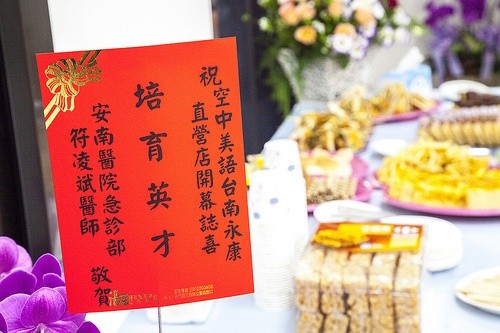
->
[247,138,310,311]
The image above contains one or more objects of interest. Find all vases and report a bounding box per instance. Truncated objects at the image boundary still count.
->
[272,45,404,104]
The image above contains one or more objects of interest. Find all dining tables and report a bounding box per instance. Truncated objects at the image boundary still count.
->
[86,86,500,333]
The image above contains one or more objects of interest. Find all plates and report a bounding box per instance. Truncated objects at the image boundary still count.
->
[313,199,396,223]
[374,98,442,122]
[438,80,493,101]
[454,265,499,315]
[369,138,490,157]
[383,185,499,217]
[379,215,471,273]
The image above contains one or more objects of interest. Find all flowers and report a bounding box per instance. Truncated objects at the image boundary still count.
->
[260,0,433,117]
[0,236,101,333]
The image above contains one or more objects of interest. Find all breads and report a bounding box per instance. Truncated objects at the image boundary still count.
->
[292,247,422,333]
